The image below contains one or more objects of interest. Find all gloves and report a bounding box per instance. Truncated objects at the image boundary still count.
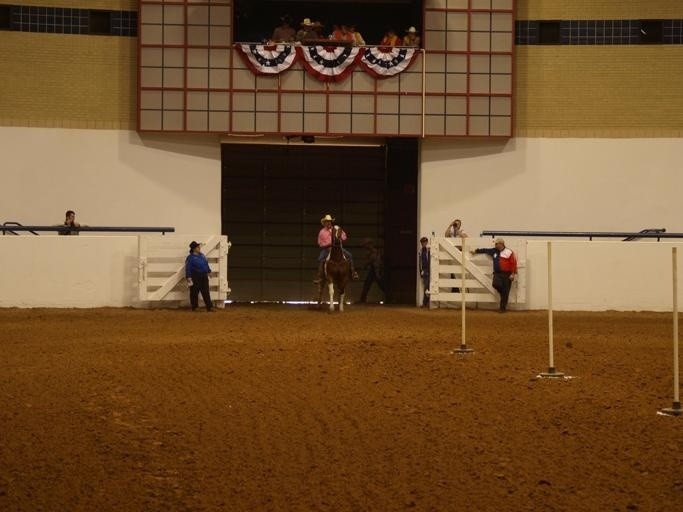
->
[509,274,515,281]
[470,248,478,255]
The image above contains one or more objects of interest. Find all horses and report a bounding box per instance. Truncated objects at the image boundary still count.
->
[317,220,350,313]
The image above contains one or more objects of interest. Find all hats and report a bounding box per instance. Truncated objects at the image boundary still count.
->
[360,238,374,246]
[494,237,505,244]
[190,241,201,253]
[321,215,336,226]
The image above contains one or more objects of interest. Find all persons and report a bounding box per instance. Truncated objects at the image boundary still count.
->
[263,19,365,46]
[469,237,518,313]
[379,28,403,46]
[444,219,468,293]
[313,215,359,283]
[185,241,216,312]
[418,236,430,309]
[358,240,393,304]
[402,26,420,46]
[57,211,81,235]
